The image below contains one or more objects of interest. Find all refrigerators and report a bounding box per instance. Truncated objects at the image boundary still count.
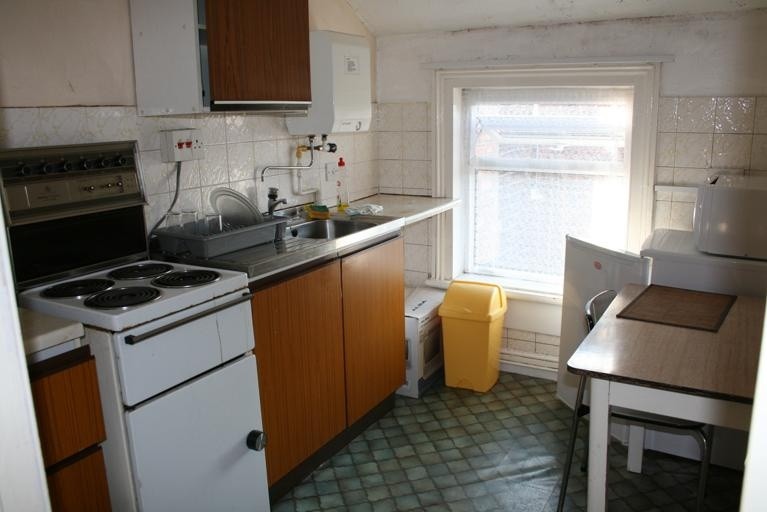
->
[555,227,767,471]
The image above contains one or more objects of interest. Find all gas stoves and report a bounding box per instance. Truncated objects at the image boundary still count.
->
[19,260,247,316]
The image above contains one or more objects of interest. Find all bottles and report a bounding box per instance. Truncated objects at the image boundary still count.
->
[336,157,349,212]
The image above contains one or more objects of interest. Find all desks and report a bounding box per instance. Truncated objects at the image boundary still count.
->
[567,282,766,511]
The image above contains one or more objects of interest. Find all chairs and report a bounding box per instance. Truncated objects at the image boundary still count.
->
[555,287,714,511]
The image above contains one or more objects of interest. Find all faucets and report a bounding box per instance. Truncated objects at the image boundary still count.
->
[267,185,287,212]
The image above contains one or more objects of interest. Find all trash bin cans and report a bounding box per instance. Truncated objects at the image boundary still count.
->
[439,279,509,393]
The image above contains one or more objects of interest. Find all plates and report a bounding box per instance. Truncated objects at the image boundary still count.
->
[209,187,264,226]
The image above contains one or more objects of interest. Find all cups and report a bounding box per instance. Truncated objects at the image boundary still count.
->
[166,208,222,235]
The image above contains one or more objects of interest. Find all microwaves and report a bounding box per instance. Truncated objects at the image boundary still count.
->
[692,174,767,260]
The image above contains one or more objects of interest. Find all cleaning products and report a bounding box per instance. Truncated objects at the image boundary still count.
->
[335,155,350,215]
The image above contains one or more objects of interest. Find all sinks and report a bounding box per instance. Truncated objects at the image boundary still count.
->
[279,208,403,240]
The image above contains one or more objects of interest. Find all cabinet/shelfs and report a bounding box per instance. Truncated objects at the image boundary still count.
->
[128,0,312,118]
[246,217,406,511]
[23,322,114,512]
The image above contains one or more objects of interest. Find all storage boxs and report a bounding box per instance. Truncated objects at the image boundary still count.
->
[394,283,448,398]
